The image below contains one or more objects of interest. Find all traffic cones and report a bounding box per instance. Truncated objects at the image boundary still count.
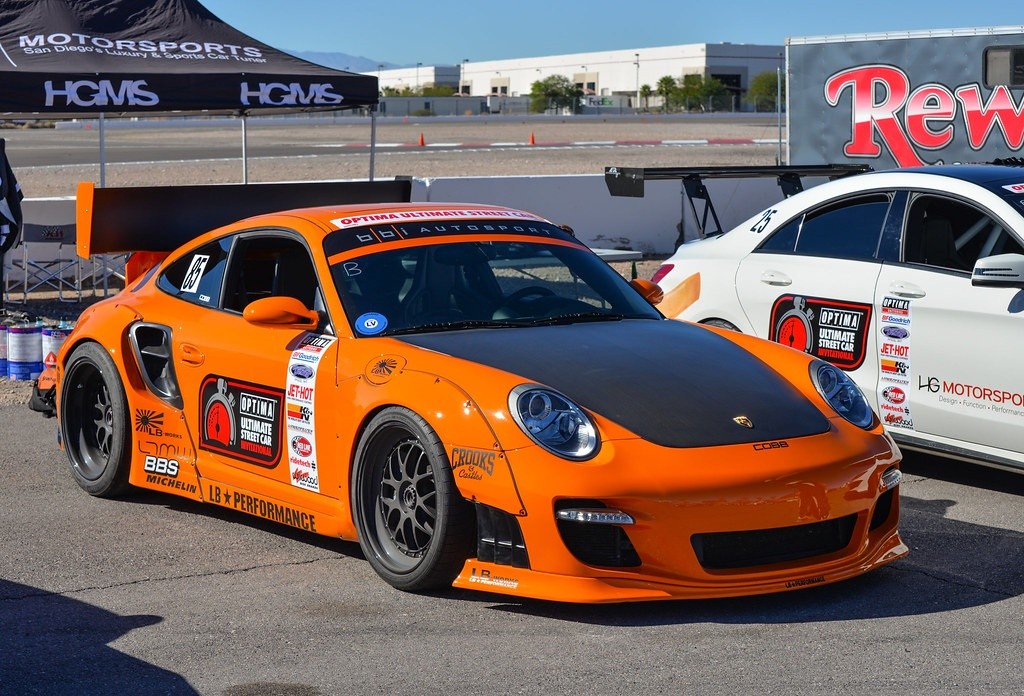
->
[528,131,536,145]
[417,132,425,146]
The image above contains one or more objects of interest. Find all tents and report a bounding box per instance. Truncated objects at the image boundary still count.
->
[0,0,380,323]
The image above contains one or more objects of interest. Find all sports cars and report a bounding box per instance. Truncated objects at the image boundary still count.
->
[650,162,1024,474]
[27,201,911,606]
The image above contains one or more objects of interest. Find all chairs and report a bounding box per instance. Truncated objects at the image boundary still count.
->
[399,242,499,327]
[271,249,319,326]
[920,212,959,268]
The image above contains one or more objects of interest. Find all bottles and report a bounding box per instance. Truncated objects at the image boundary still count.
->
[58,316,67,328]
[35,316,42,326]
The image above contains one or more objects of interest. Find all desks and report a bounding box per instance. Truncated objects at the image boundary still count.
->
[402,242,643,313]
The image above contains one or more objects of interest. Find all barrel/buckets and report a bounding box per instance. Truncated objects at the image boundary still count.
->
[7,325,42,381]
[0,323,16,378]
[42,325,75,368]
